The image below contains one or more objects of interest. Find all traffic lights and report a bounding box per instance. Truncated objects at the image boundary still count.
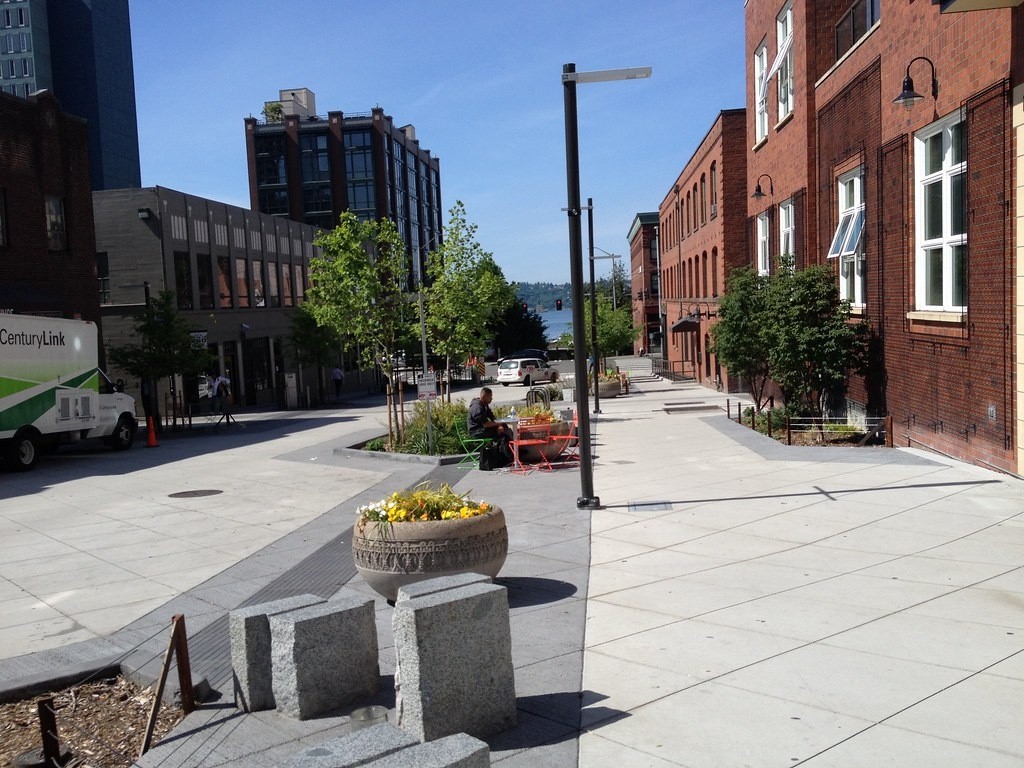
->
[556,299,562,310]
[638,289,642,301]
[522,304,527,316]
[643,288,649,300]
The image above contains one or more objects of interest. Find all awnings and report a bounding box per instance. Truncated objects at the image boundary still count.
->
[668,318,700,334]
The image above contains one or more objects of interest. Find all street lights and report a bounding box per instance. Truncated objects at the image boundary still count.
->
[587,245,623,358]
[117,280,168,435]
[557,64,655,508]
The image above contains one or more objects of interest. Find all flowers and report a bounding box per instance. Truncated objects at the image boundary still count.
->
[356,476,493,549]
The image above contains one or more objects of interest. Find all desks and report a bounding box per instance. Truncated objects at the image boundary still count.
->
[492,417,548,471]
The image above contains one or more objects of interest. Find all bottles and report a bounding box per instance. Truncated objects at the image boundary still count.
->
[511,406,515,419]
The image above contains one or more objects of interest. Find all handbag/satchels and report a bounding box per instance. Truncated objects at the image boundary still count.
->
[479,443,508,471]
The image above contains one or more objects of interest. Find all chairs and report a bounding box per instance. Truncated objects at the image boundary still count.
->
[546,410,579,469]
[454,415,496,470]
[508,417,553,476]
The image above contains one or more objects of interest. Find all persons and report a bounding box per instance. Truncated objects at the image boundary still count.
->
[332,365,345,394]
[467,388,532,465]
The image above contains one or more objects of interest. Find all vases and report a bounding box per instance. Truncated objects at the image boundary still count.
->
[352,505,508,602]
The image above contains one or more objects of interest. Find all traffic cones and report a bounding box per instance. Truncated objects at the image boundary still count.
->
[143,415,161,449]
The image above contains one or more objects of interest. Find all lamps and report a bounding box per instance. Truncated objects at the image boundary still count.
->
[677,301,716,324]
[892,58,938,111]
[750,174,773,200]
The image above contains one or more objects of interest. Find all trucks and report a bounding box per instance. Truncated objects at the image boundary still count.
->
[1,311,139,472]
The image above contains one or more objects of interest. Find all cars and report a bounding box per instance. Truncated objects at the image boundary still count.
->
[495,357,559,386]
[197,374,211,398]
[496,348,550,368]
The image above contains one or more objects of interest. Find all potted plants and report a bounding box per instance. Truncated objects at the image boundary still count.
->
[590,376,621,398]
[498,405,570,464]
